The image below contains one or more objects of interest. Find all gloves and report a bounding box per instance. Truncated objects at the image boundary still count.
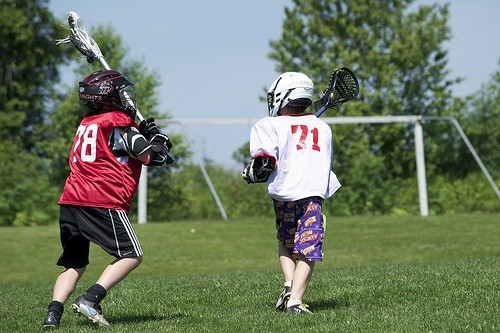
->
[242,163,255,185]
[138,117,172,153]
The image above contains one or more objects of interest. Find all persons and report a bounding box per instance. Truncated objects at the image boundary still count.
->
[242,72,341,314]
[44,70,172,329]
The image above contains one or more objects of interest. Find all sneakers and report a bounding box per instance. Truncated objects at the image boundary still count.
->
[72,293,111,330]
[287,303,314,315]
[275,286,292,312]
[42,312,59,329]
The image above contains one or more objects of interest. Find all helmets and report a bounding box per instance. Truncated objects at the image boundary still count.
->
[79,70,136,122]
[268,71,315,117]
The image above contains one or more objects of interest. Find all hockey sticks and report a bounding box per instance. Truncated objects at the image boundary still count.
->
[245,67,360,182]
[67,10,175,167]
